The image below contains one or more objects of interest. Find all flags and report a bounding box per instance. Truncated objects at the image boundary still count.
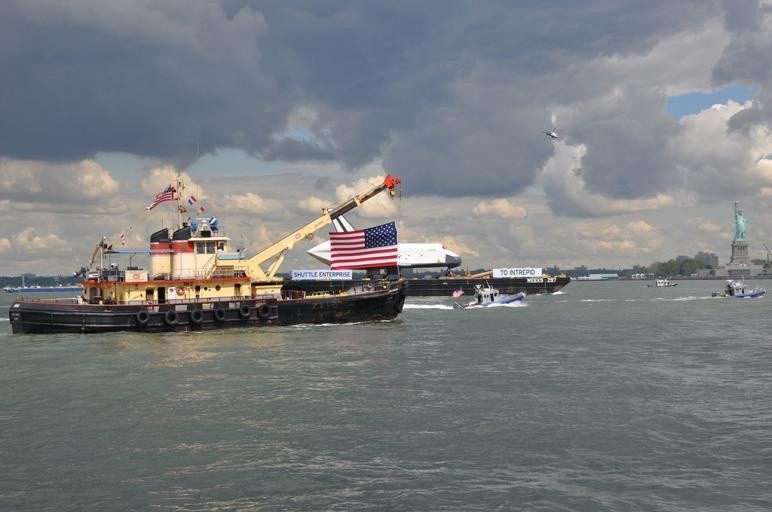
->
[197,206,206,214]
[145,183,179,211]
[188,194,197,205]
[328,222,398,269]
[452,285,464,298]
[120,227,125,246]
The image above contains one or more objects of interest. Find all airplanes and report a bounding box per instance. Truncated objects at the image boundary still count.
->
[308,206,462,267]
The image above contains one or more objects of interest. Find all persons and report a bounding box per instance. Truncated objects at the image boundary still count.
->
[733,201,750,242]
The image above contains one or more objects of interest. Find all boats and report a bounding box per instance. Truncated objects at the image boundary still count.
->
[454,284,524,310]
[712,280,765,299]
[647,280,677,288]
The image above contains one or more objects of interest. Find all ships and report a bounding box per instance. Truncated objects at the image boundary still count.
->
[8,172,407,335]
[280,267,572,294]
[3,285,83,293]
